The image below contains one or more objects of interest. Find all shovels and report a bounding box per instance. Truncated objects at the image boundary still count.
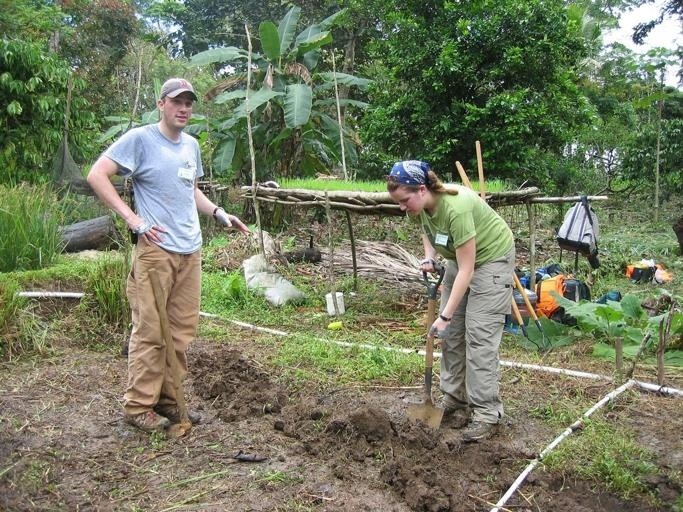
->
[402,265,446,429]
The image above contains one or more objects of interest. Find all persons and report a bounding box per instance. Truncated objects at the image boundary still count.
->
[388,160,515,442]
[87,78,251,434]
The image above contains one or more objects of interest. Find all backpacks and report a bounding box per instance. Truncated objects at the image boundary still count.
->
[556,195,600,257]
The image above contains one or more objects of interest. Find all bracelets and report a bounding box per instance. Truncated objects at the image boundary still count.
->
[441,315,452,322]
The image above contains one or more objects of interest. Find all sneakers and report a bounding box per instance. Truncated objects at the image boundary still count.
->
[155,404,202,423]
[461,422,499,441]
[439,401,467,415]
[127,411,170,434]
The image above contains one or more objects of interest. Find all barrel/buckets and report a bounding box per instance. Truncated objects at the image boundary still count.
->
[507,277,537,331]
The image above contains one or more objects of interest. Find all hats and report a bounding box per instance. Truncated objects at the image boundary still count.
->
[160,78,198,101]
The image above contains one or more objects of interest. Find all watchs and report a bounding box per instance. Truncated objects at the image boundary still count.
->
[212,206,226,221]
